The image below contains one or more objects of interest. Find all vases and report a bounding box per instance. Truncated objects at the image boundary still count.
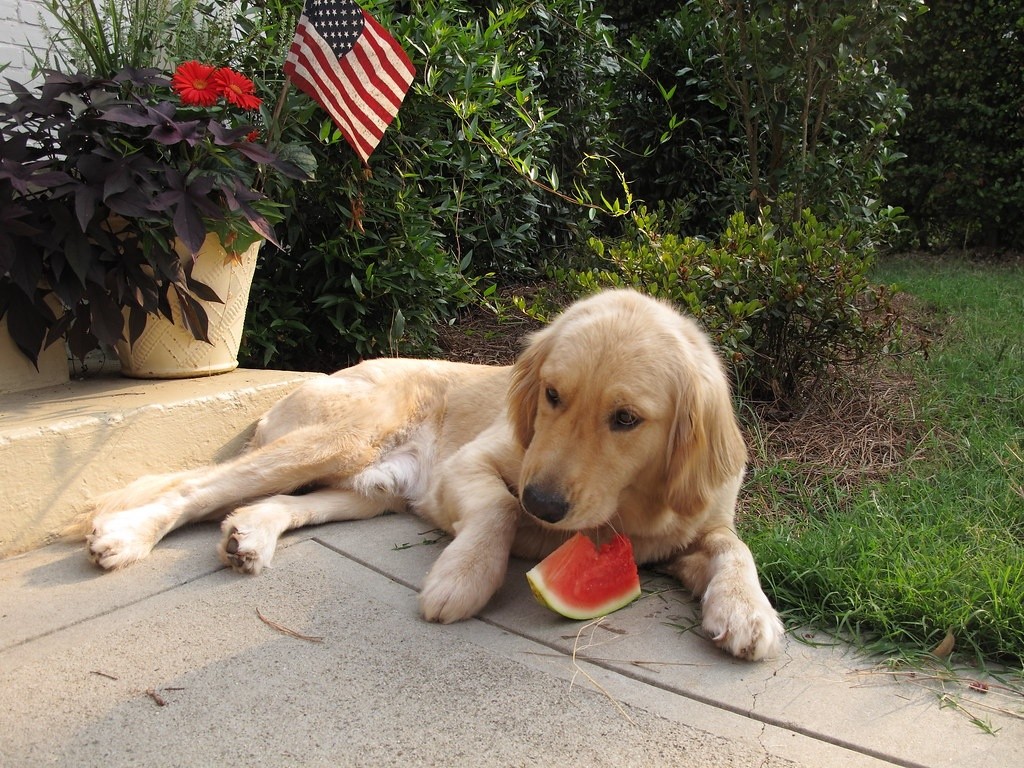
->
[104,218,269,379]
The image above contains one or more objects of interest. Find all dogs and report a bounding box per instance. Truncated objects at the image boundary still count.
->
[87,287,783,661]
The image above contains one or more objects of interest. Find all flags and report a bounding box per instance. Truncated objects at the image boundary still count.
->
[283,0,417,164]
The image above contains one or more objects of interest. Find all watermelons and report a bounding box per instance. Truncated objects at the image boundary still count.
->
[525,531,641,621]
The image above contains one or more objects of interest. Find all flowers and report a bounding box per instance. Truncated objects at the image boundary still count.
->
[0,60,317,371]
